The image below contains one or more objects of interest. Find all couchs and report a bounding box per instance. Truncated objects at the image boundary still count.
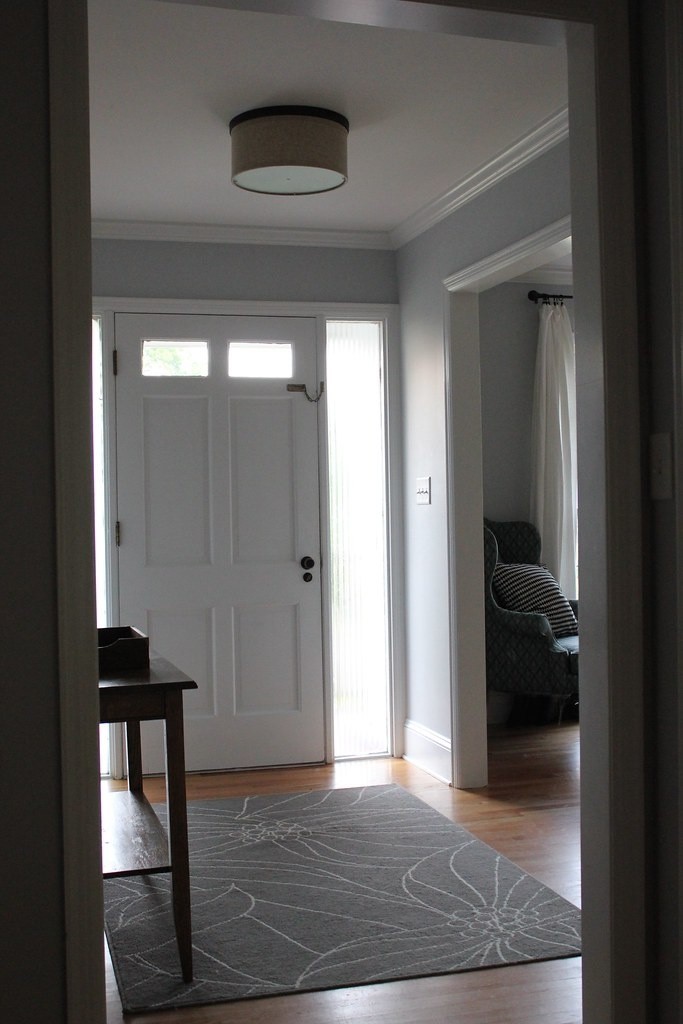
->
[480,518,580,726]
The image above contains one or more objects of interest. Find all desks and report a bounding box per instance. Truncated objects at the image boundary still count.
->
[98,647,198,981]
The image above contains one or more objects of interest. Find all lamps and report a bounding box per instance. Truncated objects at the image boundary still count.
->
[228,104,349,196]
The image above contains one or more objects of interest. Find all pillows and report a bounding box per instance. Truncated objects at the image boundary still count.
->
[491,560,580,635]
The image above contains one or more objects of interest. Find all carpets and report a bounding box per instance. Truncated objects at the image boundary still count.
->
[98,783,582,1015]
